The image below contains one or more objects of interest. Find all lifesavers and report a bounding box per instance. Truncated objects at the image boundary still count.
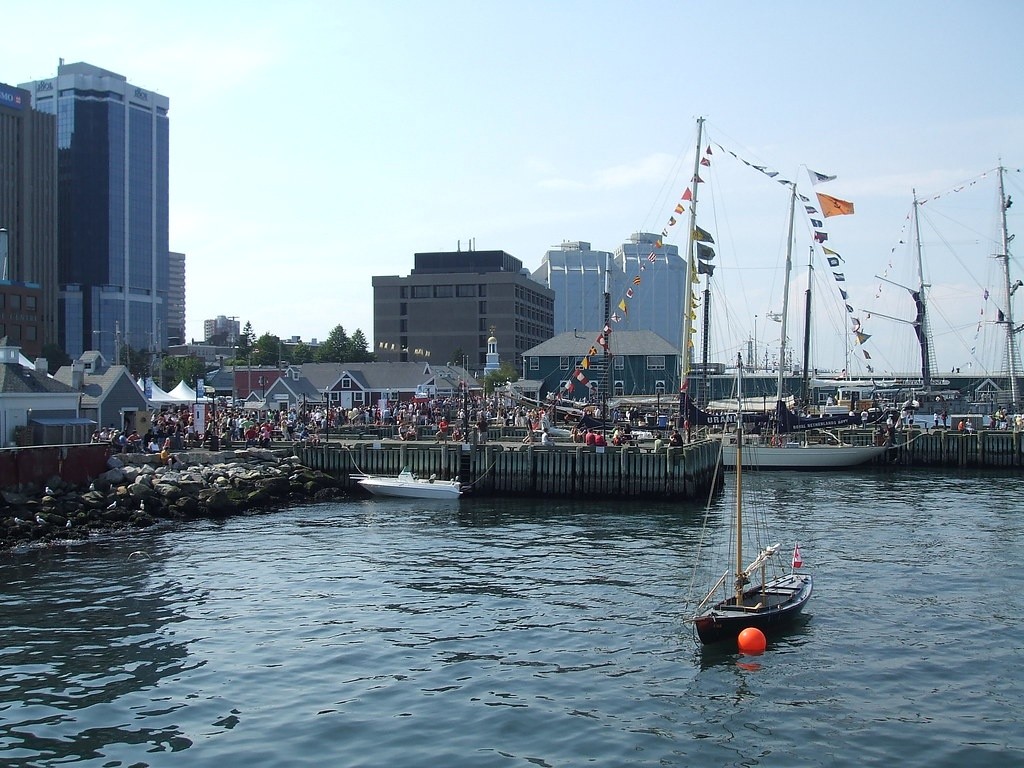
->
[771,436,782,447]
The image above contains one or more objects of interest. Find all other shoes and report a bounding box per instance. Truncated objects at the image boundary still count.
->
[435,442,439,444]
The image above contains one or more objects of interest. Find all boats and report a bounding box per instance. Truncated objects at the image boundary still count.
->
[357,467,464,500]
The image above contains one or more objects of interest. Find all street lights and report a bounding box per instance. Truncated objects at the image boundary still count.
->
[92,320,119,365]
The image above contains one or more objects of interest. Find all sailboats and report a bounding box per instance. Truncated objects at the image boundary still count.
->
[502,116,1022,470]
[680,351,814,642]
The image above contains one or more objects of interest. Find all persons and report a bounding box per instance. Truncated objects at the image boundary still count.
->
[790,394,1024,444]
[359,395,689,452]
[90,404,358,469]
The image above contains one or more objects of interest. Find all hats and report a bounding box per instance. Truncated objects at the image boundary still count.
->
[442,417,446,420]
[461,409,465,412]
[114,430,119,433]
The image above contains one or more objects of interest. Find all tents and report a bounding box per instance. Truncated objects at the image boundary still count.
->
[137,376,217,404]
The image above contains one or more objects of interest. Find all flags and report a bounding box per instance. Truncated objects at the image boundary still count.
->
[718,140,875,373]
[808,170,836,186]
[817,193,854,218]
[792,541,802,568]
[876,168,1020,367]
[555,140,715,400]
